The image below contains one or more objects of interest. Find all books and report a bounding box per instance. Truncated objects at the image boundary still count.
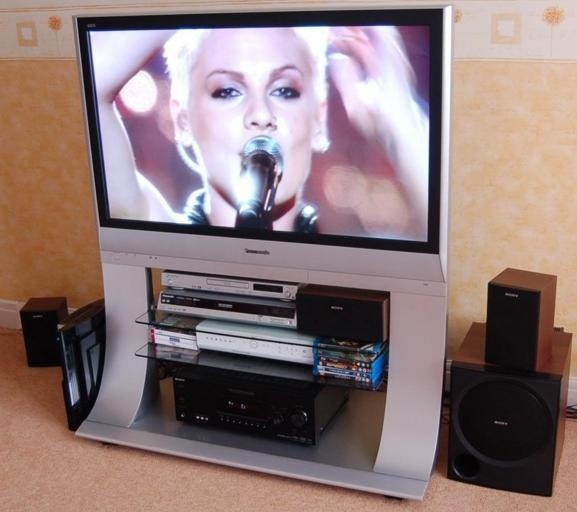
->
[312,339,389,384]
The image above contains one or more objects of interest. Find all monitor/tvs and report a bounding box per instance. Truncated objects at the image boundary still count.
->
[71,4,453,284]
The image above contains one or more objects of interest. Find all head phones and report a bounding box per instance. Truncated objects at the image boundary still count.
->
[182,186,319,235]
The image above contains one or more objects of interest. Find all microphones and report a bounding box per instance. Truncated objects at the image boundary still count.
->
[235,134,286,230]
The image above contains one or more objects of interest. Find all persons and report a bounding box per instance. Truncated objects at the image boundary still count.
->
[88,25,432,243]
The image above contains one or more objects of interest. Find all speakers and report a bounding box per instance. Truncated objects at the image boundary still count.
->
[485,267,558,372]
[19,296,68,368]
[446,321,573,497]
[295,282,390,344]
